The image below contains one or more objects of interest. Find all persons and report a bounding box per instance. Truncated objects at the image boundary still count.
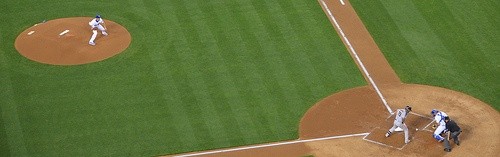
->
[89,16,109,46]
[431,110,450,142]
[385,106,412,144]
[444,116,462,152]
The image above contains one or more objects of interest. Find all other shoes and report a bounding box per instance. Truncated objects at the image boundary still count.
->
[438,138,444,143]
[385,133,389,137]
[405,140,410,143]
[444,148,451,151]
[454,142,459,145]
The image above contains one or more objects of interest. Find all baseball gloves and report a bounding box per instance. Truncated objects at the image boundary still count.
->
[93,27,98,30]
[432,123,438,130]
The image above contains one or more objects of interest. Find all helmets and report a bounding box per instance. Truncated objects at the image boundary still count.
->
[431,110,437,114]
[405,106,411,112]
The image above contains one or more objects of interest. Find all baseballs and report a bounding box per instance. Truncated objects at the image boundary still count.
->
[416,128,418,131]
